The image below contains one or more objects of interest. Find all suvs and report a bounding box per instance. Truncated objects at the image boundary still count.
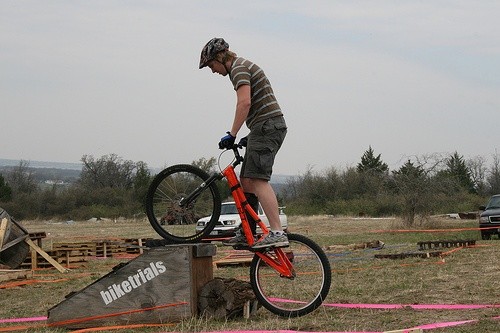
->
[196,201,287,236]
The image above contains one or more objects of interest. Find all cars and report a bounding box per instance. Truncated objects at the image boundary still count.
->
[478,194,500,239]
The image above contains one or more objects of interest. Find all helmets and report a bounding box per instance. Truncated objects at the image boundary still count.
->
[198,37,229,69]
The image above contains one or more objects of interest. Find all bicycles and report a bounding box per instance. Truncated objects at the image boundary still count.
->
[147,139,331,318]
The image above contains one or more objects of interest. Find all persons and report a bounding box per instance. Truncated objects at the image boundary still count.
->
[197,36,292,252]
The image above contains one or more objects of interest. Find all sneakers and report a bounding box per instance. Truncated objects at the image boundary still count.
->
[221,230,254,247]
[250,229,289,252]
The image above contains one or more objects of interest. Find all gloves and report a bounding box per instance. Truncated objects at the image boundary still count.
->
[238,136,248,149]
[218,131,236,150]
